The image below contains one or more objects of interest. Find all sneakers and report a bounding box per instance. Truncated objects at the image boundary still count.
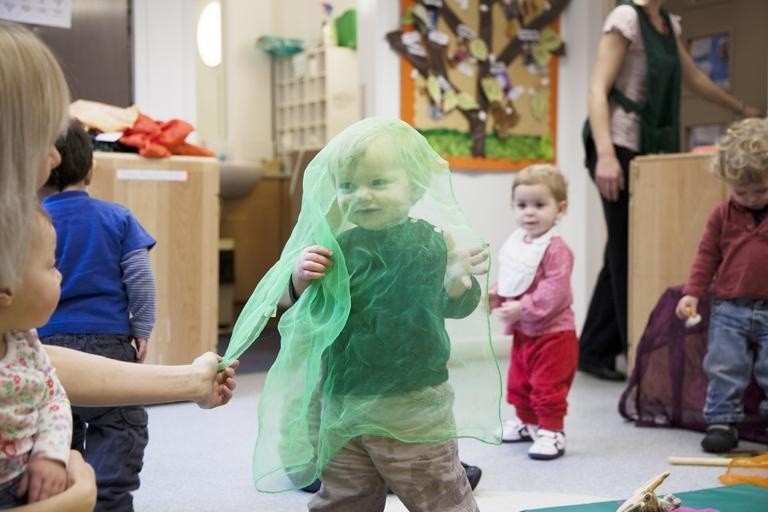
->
[502,416,564,460]
[701,424,739,453]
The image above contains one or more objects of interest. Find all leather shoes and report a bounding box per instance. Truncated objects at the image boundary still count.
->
[580,365,627,380]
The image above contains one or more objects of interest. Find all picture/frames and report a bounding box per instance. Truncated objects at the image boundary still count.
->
[680,27,732,97]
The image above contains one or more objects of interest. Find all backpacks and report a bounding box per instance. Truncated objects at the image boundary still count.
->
[619,285,707,429]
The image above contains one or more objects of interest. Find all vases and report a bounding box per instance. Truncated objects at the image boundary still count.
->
[322,24,339,47]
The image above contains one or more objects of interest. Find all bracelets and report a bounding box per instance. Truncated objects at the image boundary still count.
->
[286,274,299,303]
[738,101,747,116]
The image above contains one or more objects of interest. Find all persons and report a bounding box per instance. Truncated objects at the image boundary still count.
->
[675,117,767,452]
[0,203,73,511]
[1,22,238,511]
[263,114,492,511]
[37,117,158,511]
[483,162,579,459]
[576,0,766,382]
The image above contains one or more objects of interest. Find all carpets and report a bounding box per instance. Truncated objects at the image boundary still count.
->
[518,483,768,512]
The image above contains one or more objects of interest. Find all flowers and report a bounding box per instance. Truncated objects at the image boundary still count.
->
[321,1,334,23]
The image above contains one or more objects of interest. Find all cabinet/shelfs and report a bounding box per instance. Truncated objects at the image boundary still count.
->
[270,47,362,159]
[92,151,287,366]
[627,149,737,385]
